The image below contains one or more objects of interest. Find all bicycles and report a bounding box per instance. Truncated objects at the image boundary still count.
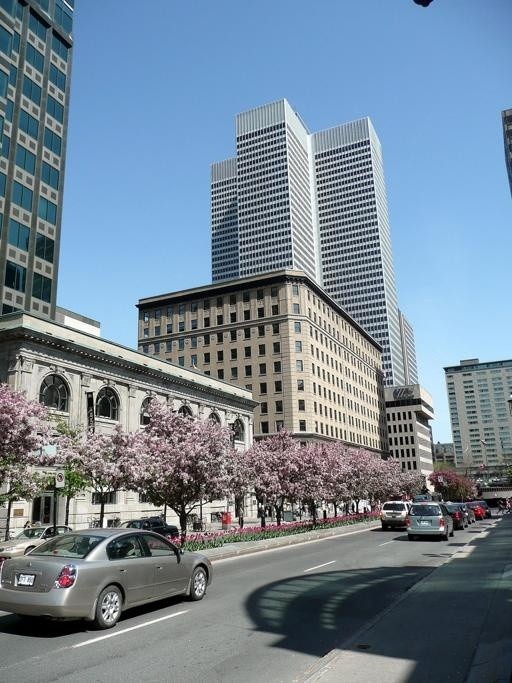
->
[185,513,212,532]
[86,515,122,528]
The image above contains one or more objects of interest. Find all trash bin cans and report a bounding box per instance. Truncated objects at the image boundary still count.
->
[107,519,121,528]
[193,522,201,532]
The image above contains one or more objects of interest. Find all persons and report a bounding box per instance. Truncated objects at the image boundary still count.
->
[24,521,30,528]
[496,497,512,514]
[239,507,244,528]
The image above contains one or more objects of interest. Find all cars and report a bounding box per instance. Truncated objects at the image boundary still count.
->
[470,475,510,488]
[1,524,71,560]
[114,518,179,540]
[1,528,215,630]
[379,495,491,541]
